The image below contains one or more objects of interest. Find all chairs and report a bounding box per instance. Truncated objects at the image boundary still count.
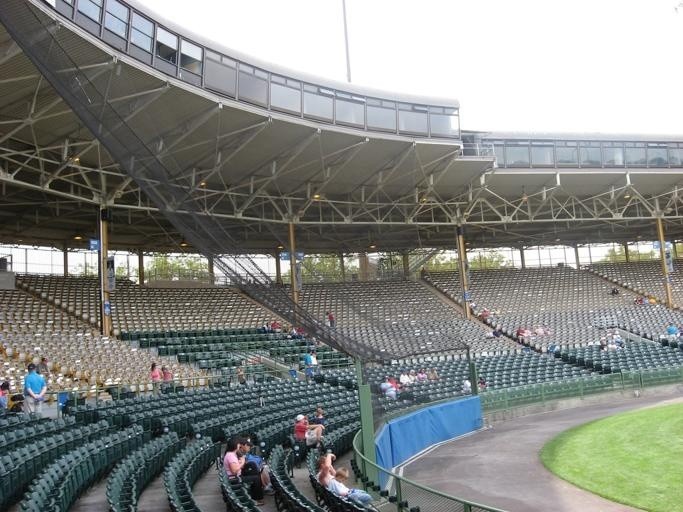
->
[0,275,416,512]
[416,275,683,408]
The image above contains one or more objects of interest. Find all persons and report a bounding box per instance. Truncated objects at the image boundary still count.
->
[462,377,472,395]
[235,366,249,386]
[611,286,620,296]
[106,261,115,278]
[0,381,10,410]
[264,309,337,376]
[293,404,374,509]
[599,330,627,353]
[37,357,50,376]
[146,363,165,395]
[22,363,48,416]
[634,294,657,306]
[664,250,674,280]
[379,366,441,401]
[666,322,679,336]
[221,432,278,507]
[477,377,488,392]
[677,323,683,336]
[469,297,553,343]
[160,365,174,390]
